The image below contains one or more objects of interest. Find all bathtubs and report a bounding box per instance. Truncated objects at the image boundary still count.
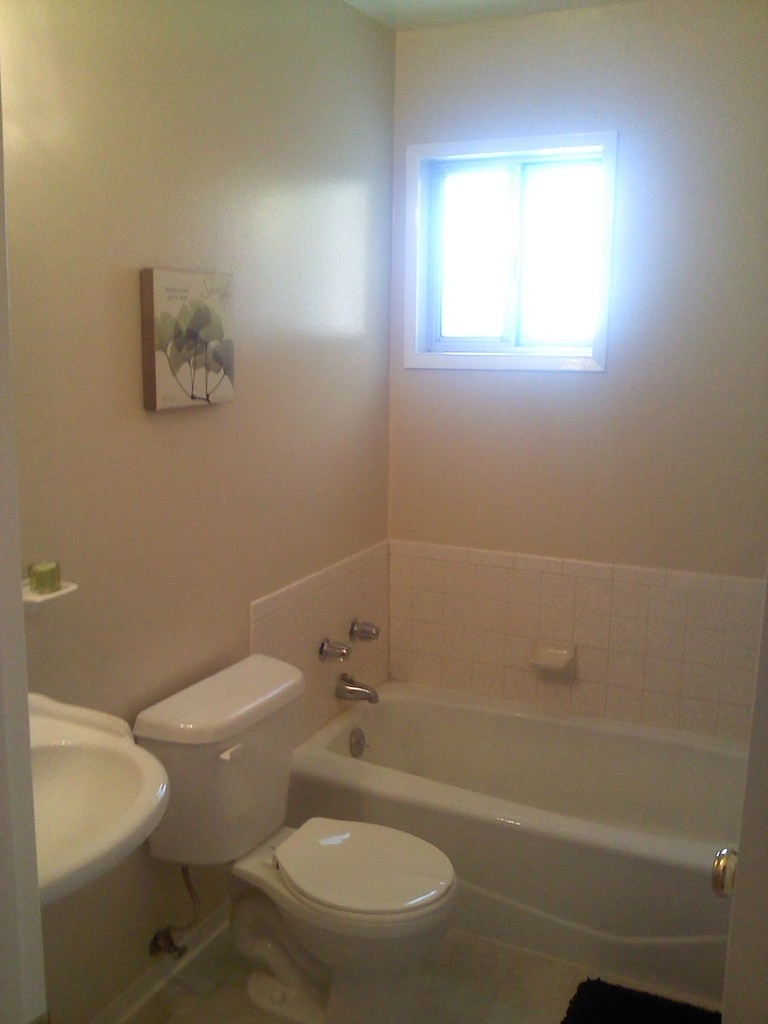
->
[285,679,749,1003]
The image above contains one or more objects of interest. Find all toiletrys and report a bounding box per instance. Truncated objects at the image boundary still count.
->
[28,559,62,594]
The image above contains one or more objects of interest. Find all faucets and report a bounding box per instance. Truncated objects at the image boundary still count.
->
[336,671,379,704]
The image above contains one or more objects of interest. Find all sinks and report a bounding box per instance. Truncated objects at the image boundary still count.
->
[26,687,171,909]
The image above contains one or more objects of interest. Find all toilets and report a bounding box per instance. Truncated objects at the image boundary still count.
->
[129,650,461,1023]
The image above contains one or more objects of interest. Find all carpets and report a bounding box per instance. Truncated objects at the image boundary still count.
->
[557,973,723,1024]
